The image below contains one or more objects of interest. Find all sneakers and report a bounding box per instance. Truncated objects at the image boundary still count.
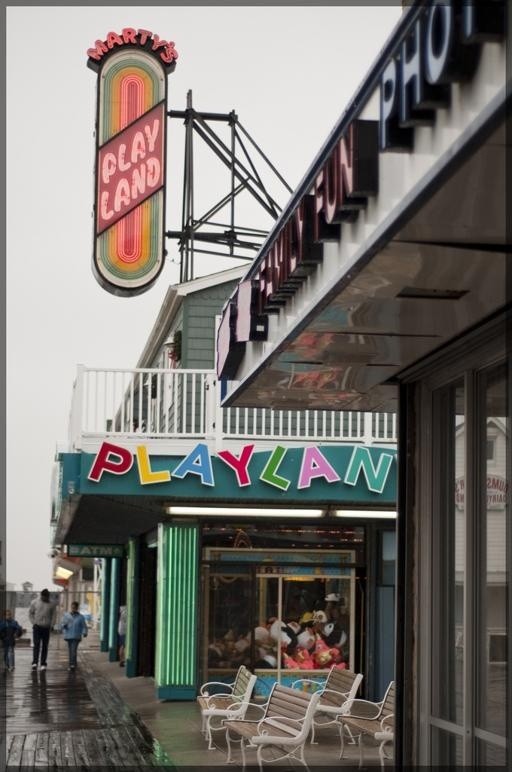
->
[4,664,75,672]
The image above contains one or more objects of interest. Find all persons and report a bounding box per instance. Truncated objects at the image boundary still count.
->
[117,597,128,667]
[60,600,88,672]
[29,589,57,672]
[0,608,23,672]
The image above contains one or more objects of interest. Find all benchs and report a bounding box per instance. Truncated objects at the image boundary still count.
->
[198,663,394,771]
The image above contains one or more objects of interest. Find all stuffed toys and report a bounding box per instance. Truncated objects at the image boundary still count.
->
[208,593,351,672]
